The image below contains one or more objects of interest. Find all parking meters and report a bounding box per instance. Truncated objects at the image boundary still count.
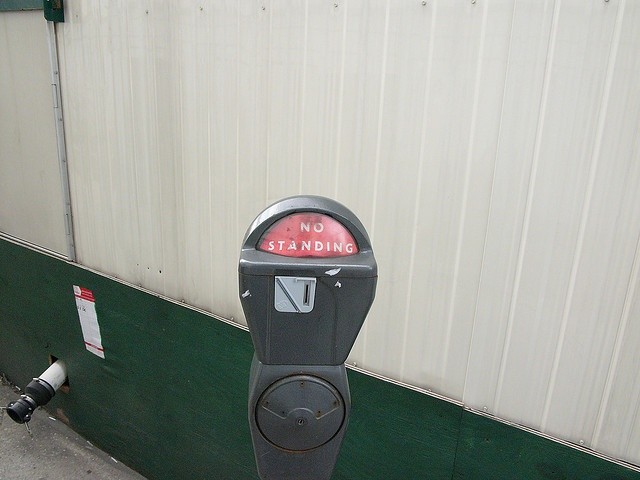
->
[239,195,378,479]
